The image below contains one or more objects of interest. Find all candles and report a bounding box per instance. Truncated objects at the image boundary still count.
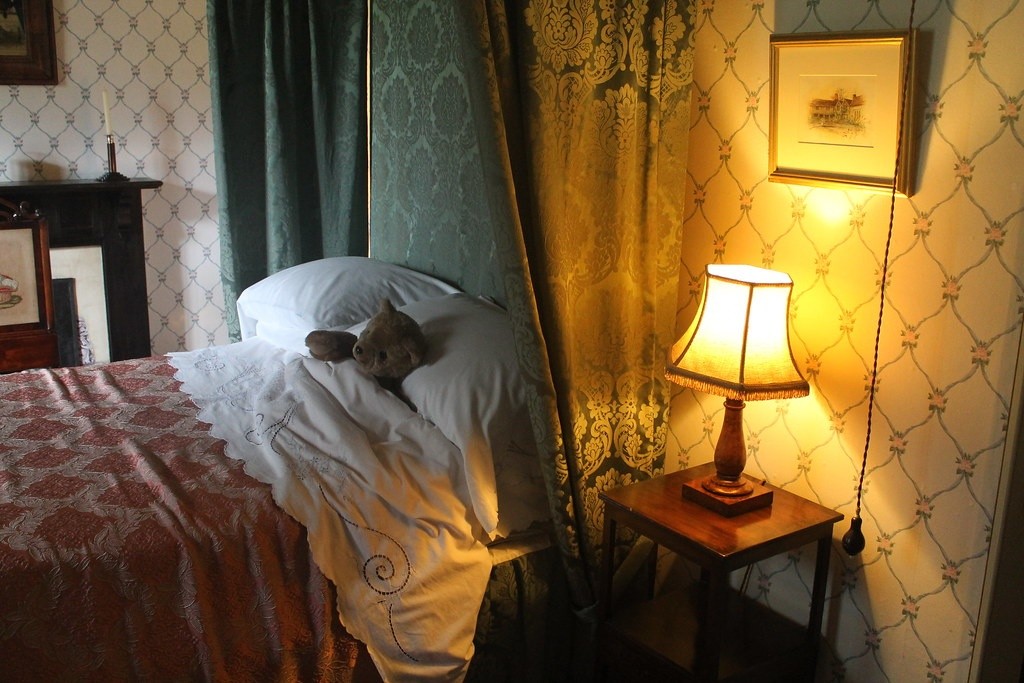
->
[102,88,112,136]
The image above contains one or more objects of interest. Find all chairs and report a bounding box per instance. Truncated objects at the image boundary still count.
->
[0,199,83,375]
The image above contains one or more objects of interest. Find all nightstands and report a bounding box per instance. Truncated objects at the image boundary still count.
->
[597,461,844,683]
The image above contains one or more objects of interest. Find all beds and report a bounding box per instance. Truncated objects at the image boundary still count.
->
[1,348,565,683]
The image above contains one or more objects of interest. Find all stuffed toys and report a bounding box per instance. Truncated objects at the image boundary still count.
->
[305,297,426,395]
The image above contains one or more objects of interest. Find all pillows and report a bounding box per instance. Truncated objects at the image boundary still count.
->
[342,291,553,541]
[239,256,448,329]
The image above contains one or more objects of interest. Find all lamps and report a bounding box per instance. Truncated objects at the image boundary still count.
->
[663,263,810,518]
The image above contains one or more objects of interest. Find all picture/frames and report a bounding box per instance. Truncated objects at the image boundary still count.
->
[0,0,59,86]
[767,29,920,199]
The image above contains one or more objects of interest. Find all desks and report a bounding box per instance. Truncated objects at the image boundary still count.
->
[0,176,163,363]
